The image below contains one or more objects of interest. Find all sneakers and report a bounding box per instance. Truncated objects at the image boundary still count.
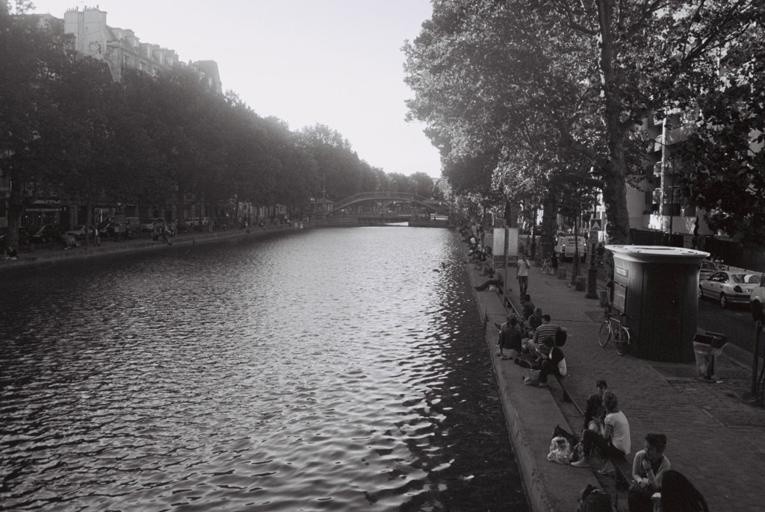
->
[597,459,615,475]
[571,456,591,468]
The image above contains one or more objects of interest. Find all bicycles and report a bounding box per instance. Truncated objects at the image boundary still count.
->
[597,306,632,359]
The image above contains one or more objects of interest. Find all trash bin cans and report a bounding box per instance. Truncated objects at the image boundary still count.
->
[692,330,728,382]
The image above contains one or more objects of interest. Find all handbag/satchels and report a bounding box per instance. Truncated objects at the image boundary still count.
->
[547,426,579,464]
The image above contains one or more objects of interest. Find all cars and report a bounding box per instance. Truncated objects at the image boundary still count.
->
[696,260,765,323]
[20,211,303,251]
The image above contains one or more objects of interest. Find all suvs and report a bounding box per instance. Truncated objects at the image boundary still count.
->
[553,233,586,263]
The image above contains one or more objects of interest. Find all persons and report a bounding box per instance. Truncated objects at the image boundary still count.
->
[567,379,631,467]
[515,254,531,295]
[462,219,495,269]
[476,267,504,290]
[495,294,568,387]
[629,432,712,511]
[550,253,559,271]
